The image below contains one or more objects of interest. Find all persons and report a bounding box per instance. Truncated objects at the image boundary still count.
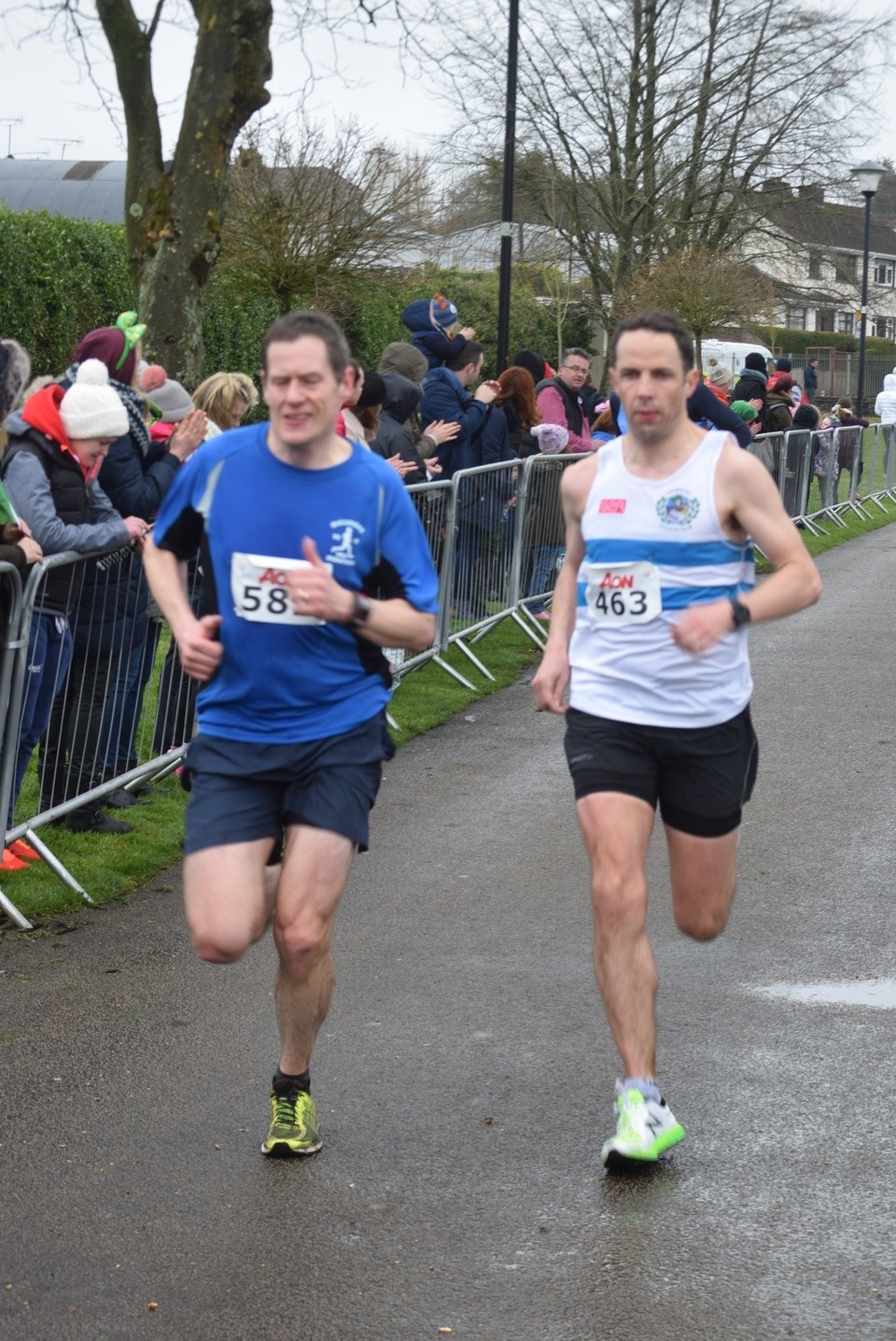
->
[1,301,870,874]
[874,367,896,478]
[530,311,822,1170]
[142,312,439,1157]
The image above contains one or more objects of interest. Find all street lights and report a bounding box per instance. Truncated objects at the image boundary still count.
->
[851,158,891,417]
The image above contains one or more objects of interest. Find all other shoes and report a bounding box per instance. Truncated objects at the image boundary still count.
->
[4,830,41,860]
[847,491,863,502]
[473,612,494,619]
[531,609,551,620]
[486,594,506,602]
[105,745,190,807]
[0,848,31,870]
[449,606,460,618]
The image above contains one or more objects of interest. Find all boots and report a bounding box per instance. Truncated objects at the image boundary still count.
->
[37,763,67,828]
[64,763,132,834]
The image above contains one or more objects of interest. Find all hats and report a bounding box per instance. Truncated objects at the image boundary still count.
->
[58,357,129,439]
[729,400,758,420]
[431,292,459,330]
[774,379,797,392]
[745,353,766,372]
[138,364,197,422]
[531,423,570,454]
[72,327,137,384]
[0,339,30,424]
[707,358,733,385]
[776,358,792,373]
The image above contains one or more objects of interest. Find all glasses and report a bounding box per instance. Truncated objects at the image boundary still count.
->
[562,364,589,375]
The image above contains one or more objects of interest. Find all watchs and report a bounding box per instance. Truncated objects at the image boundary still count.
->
[344,593,372,633]
[730,597,752,629]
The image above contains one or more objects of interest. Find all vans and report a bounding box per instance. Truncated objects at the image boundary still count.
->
[691,339,775,385]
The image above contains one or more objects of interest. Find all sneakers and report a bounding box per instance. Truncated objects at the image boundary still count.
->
[259,1072,323,1155]
[600,1087,685,1170]
